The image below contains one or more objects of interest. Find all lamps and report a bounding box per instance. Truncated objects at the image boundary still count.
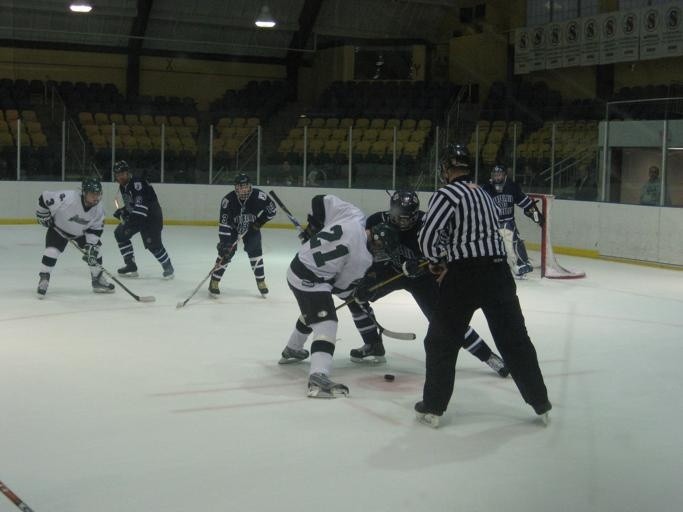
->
[69,0,92,13]
[254,0,276,27]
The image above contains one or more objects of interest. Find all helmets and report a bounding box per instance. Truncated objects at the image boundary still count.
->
[114,160,129,172]
[82,176,103,192]
[489,165,508,191]
[389,190,420,231]
[371,223,398,262]
[232,173,252,200]
[438,144,473,182]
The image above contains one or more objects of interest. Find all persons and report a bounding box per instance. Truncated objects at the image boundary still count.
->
[279,194,401,393]
[111,160,175,278]
[480,164,544,276]
[524,164,544,194]
[416,142,554,419]
[346,187,508,376]
[306,158,327,188]
[575,164,598,202]
[640,166,670,204]
[208,173,278,295]
[35,176,115,297]
[274,161,299,186]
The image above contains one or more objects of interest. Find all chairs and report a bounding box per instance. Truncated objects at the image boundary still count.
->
[0,81,682,184]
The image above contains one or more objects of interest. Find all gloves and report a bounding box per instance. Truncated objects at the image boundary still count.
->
[297,221,325,245]
[112,208,128,219]
[247,222,258,235]
[121,223,137,239]
[402,258,422,277]
[353,285,376,304]
[218,241,237,260]
[85,250,98,266]
[37,215,54,227]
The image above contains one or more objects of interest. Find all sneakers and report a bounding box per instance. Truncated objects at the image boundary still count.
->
[533,399,552,414]
[257,279,269,293]
[209,278,220,293]
[282,346,309,359]
[307,373,349,393]
[414,399,442,416]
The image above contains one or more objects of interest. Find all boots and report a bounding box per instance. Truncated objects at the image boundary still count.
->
[91,271,115,290]
[117,256,137,273]
[487,354,508,377]
[38,272,50,294]
[162,258,174,276]
[350,328,387,359]
